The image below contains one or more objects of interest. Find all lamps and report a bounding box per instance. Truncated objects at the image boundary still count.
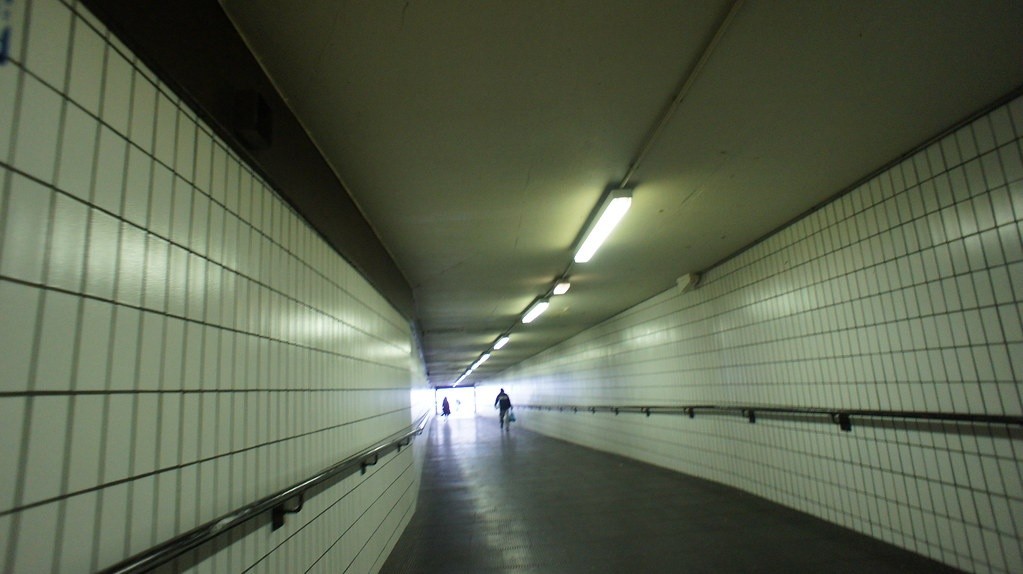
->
[451,189,635,388]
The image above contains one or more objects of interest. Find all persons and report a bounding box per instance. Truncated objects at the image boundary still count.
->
[442,397,449,416]
[495,389,512,428]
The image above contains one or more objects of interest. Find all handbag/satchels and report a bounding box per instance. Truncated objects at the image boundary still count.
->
[508,412,515,422]
